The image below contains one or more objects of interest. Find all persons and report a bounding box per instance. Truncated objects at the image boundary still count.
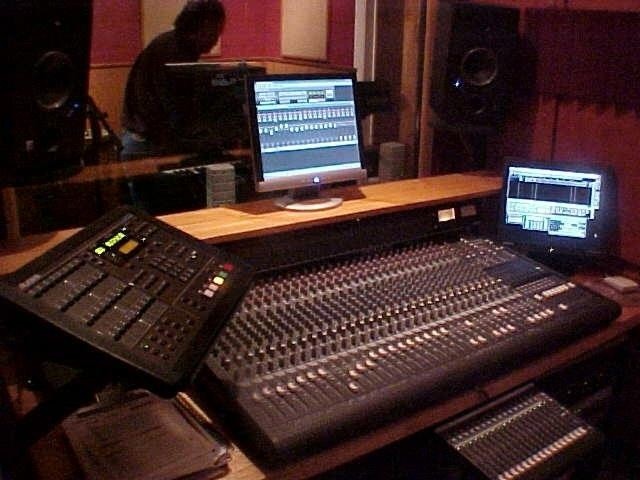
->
[116,0,227,219]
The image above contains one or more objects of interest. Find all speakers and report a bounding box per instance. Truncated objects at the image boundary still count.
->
[430,3,520,123]
[0,0,91,183]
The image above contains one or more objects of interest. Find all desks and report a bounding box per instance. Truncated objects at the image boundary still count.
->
[0,169,639,480]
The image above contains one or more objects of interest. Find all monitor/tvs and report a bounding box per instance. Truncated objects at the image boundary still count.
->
[159,63,264,173]
[494,156,616,275]
[245,69,362,210]
